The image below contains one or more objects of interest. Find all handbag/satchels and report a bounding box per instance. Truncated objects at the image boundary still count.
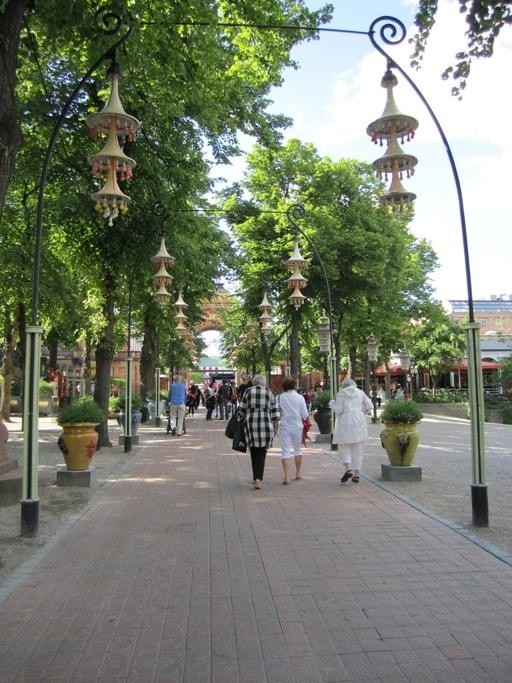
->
[224,412,248,453]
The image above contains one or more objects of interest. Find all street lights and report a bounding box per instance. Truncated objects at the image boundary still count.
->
[398,346,410,398]
[122,220,199,368]
[284,203,338,357]
[228,286,289,367]
[366,14,487,323]
[365,332,378,417]
[22,6,144,324]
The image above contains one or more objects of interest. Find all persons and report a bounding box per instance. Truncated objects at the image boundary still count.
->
[367,382,405,407]
[275,379,308,485]
[165,375,253,436]
[299,382,323,417]
[238,375,281,489]
[332,377,374,483]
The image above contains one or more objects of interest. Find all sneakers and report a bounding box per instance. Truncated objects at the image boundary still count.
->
[251,478,265,489]
[171,428,176,436]
[340,468,362,484]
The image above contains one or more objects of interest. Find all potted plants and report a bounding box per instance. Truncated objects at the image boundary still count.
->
[313,389,332,434]
[56,393,107,470]
[378,398,423,466]
[115,388,168,436]
